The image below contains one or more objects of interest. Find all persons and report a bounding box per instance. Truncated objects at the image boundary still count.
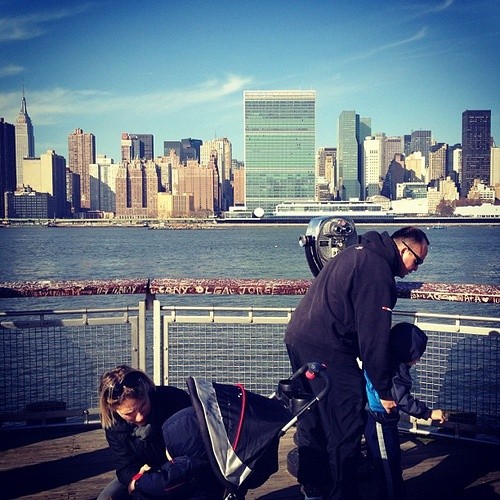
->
[361,321,449,500]
[94,365,218,500]
[284,225,431,500]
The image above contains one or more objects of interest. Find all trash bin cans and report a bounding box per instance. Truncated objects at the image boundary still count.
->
[25,401,68,425]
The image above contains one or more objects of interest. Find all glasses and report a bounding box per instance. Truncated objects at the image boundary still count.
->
[106,372,142,400]
[402,241,423,265]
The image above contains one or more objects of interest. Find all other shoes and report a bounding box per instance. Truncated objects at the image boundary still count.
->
[301,485,324,500]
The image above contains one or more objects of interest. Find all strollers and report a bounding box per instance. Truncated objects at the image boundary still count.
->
[97,360,333,500]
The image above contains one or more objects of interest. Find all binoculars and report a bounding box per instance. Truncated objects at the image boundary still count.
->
[323,218,354,258]
